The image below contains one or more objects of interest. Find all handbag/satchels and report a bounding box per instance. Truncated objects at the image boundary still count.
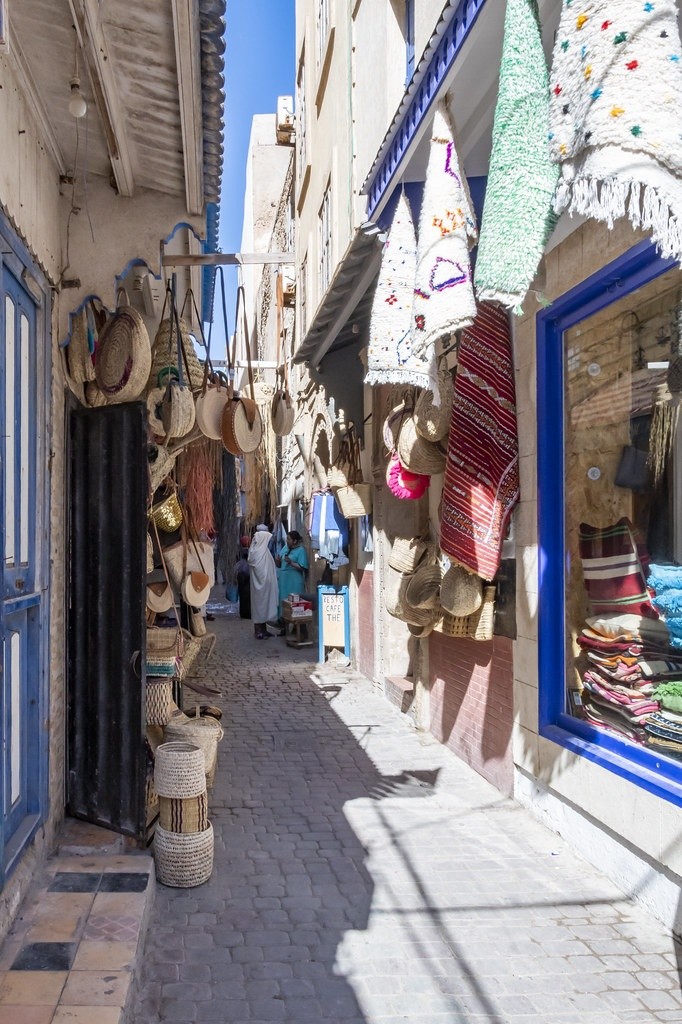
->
[65,286,295,454]
[181,571,210,607]
[162,503,216,593]
[225,582,239,604]
[327,432,372,519]
[147,581,174,613]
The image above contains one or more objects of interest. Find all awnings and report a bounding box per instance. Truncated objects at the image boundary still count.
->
[294,1,555,381]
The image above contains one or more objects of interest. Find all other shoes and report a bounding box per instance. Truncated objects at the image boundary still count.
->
[254,630,274,640]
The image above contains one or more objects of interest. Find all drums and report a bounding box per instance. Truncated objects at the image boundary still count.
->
[196,385,234,441]
[272,390,295,436]
[219,398,263,456]
[180,574,211,606]
[146,584,174,613]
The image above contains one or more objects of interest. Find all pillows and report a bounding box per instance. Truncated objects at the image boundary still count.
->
[577,516,659,620]
[584,613,668,642]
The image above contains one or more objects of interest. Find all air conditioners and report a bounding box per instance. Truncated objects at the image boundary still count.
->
[278,265,295,305]
[276,96,293,143]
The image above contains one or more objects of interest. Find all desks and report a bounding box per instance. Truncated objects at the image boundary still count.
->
[281,617,314,646]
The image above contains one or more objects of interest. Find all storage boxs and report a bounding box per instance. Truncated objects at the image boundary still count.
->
[282,598,313,618]
[288,594,299,602]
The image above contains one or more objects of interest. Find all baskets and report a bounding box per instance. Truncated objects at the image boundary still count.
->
[148,493,183,532]
[145,634,226,889]
[384,532,497,642]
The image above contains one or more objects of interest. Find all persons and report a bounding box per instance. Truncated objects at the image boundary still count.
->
[274,531,308,636]
[248,523,277,639]
[237,536,252,620]
